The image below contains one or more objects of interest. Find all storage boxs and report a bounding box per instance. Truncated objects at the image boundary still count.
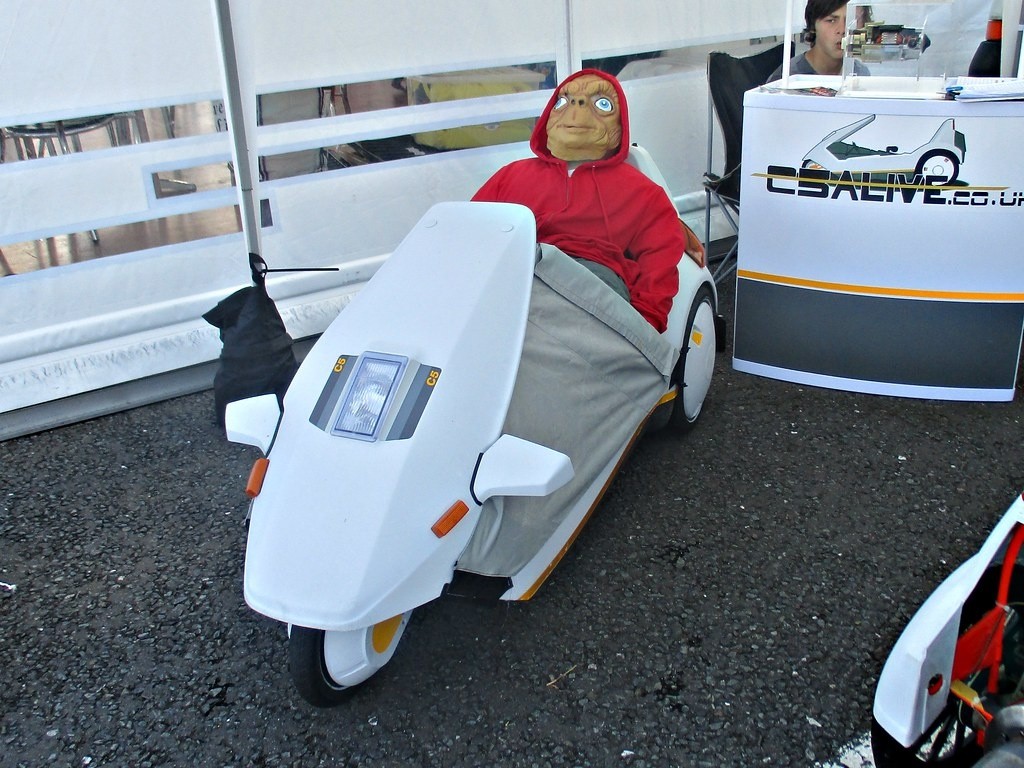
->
[405,65,548,149]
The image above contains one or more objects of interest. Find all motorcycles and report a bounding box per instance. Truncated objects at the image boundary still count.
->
[867,479,1023,768]
[222,138,729,709]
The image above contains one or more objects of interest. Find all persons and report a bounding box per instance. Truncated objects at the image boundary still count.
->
[765,0,875,84]
[454,68,687,577]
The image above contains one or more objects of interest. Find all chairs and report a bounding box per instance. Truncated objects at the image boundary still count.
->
[625,145,681,219]
[4,112,144,242]
[702,40,796,287]
